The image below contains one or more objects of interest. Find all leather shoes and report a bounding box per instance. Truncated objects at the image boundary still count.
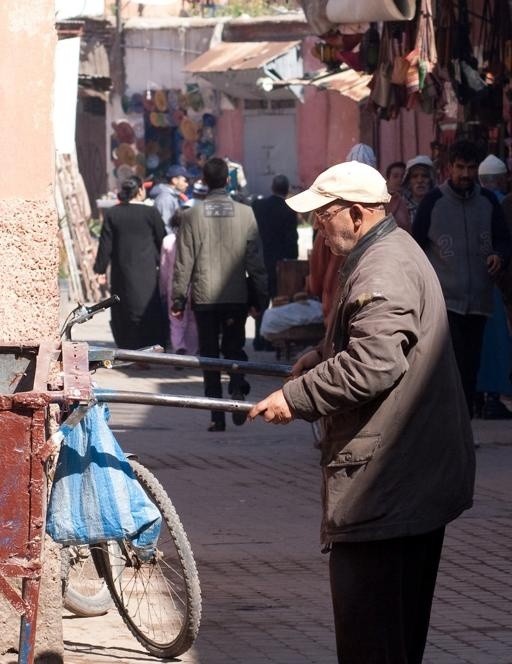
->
[232,390,246,426]
[208,422,226,432]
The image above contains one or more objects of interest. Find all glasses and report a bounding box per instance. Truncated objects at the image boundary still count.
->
[314,206,374,223]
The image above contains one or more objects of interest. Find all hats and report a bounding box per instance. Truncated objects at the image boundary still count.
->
[192,179,208,195]
[400,155,433,185]
[478,153,507,176]
[346,142,376,167]
[284,159,391,212]
[117,175,142,200]
[166,165,195,178]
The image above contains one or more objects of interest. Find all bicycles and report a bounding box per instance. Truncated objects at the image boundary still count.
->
[61,293,203,659]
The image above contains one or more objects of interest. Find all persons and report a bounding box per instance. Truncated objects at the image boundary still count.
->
[250,175,298,342]
[492,193,512,334]
[386,162,405,189]
[93,174,168,352]
[155,165,200,370]
[193,152,247,198]
[304,143,410,329]
[171,157,269,432]
[411,141,501,396]
[248,161,477,664]
[184,179,208,208]
[400,155,435,225]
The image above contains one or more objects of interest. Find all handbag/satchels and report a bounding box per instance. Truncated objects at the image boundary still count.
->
[306,0,503,142]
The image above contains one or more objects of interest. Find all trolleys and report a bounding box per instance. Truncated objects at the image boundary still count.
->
[0,338,295,664]
